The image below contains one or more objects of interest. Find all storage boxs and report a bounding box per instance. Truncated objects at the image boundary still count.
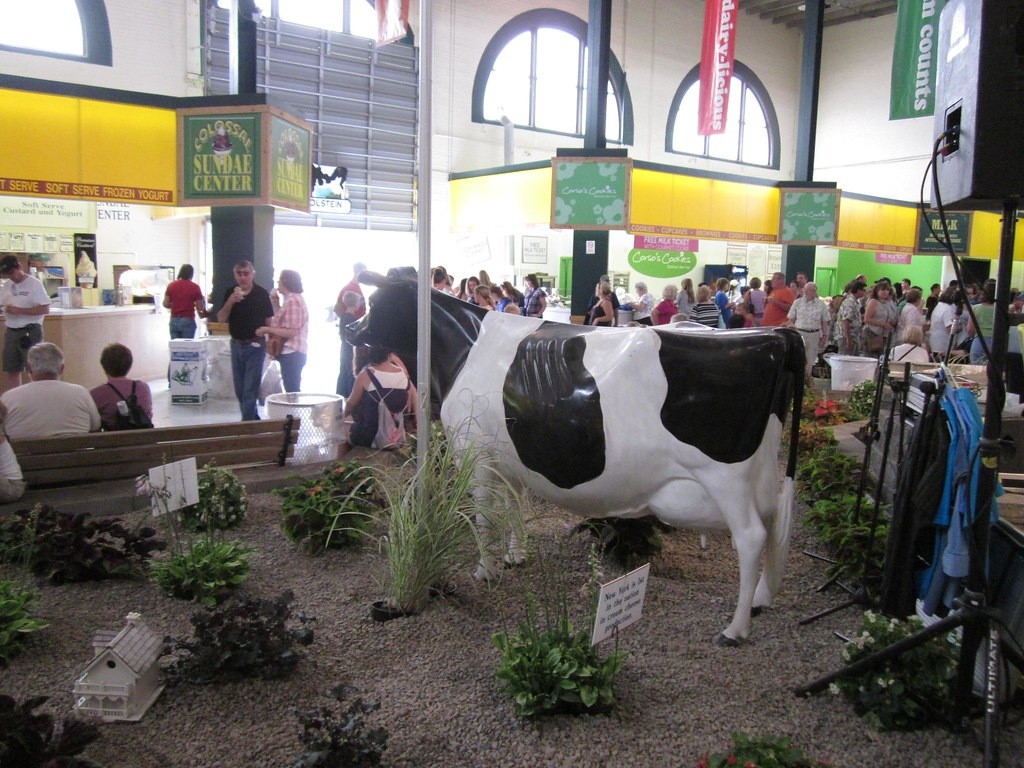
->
[907,370,979,414]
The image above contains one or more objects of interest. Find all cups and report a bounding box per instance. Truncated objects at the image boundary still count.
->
[762,297,772,303]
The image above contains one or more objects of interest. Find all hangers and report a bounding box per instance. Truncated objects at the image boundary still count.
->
[933,363,961,439]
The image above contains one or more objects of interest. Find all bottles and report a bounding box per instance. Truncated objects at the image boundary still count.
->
[116,284,123,306]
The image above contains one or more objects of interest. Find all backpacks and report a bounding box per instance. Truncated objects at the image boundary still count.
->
[362,369,410,449]
[102,380,154,430]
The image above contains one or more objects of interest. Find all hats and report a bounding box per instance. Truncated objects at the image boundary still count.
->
[0,256,18,273]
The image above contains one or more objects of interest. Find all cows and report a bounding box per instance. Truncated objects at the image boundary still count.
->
[311,163,350,199]
[344,266,807,645]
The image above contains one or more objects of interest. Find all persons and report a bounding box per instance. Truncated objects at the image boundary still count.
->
[0,400,26,501]
[650,284,680,325]
[89,343,153,432]
[686,283,725,328]
[586,274,620,327]
[834,273,930,362]
[715,272,795,328]
[256,269,308,392]
[344,345,409,452]
[789,272,831,386]
[0,255,50,392]
[333,262,369,397]
[624,281,654,326]
[162,263,205,388]
[216,260,275,421]
[431,266,547,319]
[0,342,101,438]
[674,277,697,315]
[926,278,1024,365]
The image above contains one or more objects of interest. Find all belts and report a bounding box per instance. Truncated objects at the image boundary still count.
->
[230,336,259,344]
[798,328,820,334]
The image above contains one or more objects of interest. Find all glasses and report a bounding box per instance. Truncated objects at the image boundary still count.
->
[860,289,866,292]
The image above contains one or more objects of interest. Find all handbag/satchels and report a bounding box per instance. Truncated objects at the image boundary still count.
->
[866,334,883,354]
[520,307,526,316]
[258,361,283,406]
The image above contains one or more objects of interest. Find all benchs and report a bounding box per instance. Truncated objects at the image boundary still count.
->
[10,416,301,490]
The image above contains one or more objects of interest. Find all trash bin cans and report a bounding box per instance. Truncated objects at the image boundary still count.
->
[830,357,878,390]
[264,393,344,466]
